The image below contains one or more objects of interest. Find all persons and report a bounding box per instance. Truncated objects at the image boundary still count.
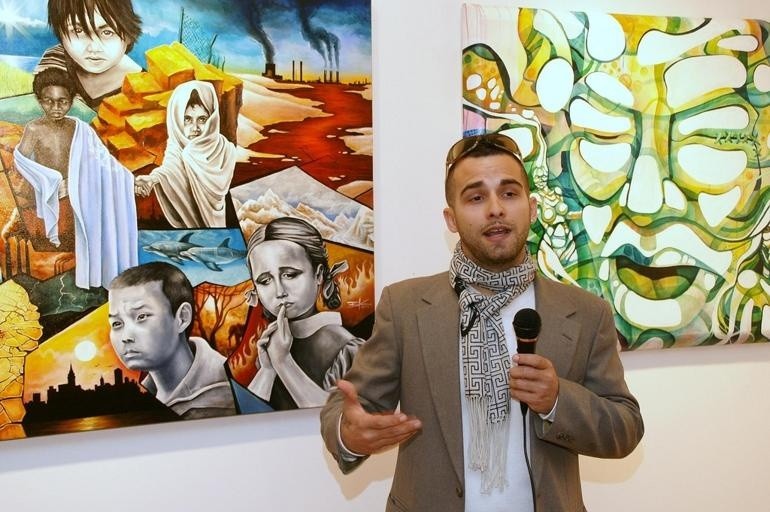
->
[319,135,644,512]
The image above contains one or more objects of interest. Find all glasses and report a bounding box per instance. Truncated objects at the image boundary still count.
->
[446,134,527,189]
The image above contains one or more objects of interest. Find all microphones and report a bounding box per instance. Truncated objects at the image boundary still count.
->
[511,307,543,418]
